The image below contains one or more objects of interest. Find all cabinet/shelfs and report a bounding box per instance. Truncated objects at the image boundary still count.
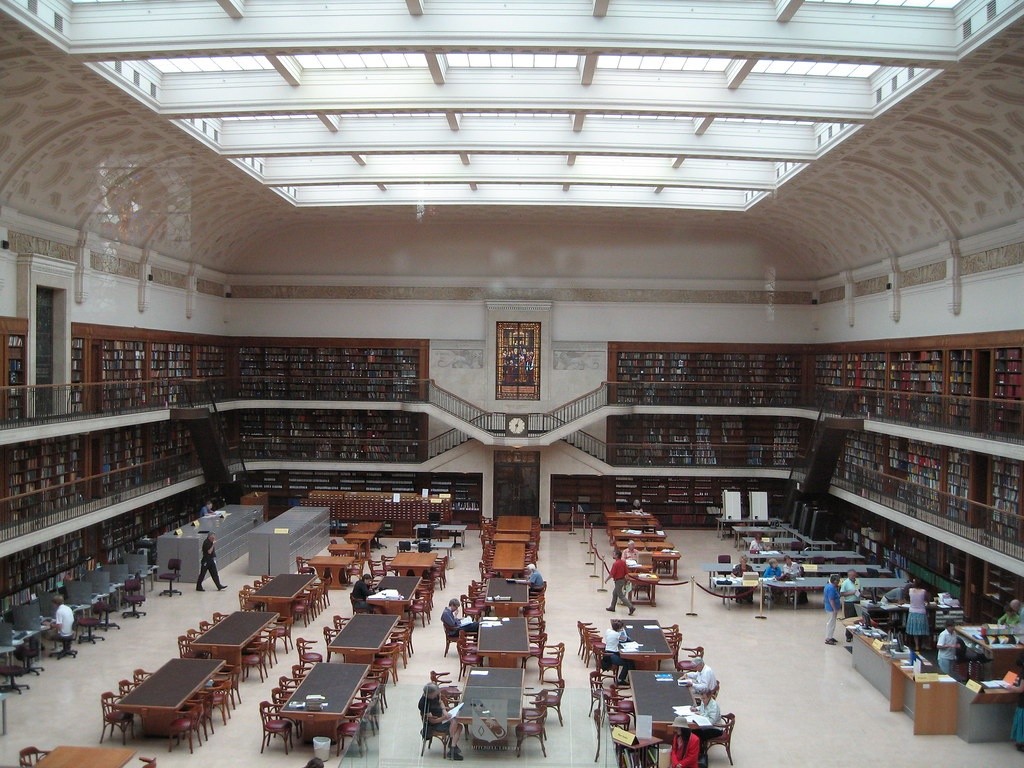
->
[247,506,330,577]
[241,492,269,523]
[550,472,791,530]
[156,504,265,581]
[0,482,224,620]
[233,470,485,539]
[828,493,1024,625]
[607,328,1024,550]
[861,602,965,651]
[0,315,429,530]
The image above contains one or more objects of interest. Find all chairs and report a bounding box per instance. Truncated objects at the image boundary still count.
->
[0,516,564,768]
[577,542,880,768]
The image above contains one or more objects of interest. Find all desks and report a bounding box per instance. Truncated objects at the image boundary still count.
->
[350,522,384,549]
[390,552,438,577]
[491,543,525,578]
[365,576,423,617]
[327,614,401,665]
[279,662,372,746]
[604,512,681,608]
[307,556,355,590]
[627,670,701,746]
[955,626,1024,681]
[250,574,318,623]
[610,619,674,671]
[345,533,374,560]
[115,658,226,738]
[31,746,136,768]
[496,516,532,535]
[492,534,530,544]
[190,610,281,667]
[0,631,40,666]
[477,616,530,668]
[484,578,529,617]
[456,667,525,750]
[702,517,1024,744]
[327,544,358,557]
[394,540,454,570]
[413,525,467,551]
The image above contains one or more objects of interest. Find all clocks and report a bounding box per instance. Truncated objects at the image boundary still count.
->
[505,414,528,438]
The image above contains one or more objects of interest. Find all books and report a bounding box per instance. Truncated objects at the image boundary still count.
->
[239,409,418,460]
[813,348,1023,539]
[245,471,414,492]
[0,435,85,528]
[103,422,192,496]
[431,475,480,510]
[0,533,96,612]
[103,506,147,562]
[234,347,419,400]
[616,446,801,503]
[211,412,230,449]
[7,334,225,422]
[147,485,220,539]
[616,352,800,405]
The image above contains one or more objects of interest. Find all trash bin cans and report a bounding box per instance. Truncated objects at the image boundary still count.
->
[312,737,331,762]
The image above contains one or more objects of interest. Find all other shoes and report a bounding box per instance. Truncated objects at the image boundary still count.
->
[448,751,463,760]
[218,585,227,590]
[825,640,836,645]
[830,638,838,643]
[846,638,851,642]
[196,588,205,591]
[629,607,636,615]
[606,607,615,612]
[448,741,461,753]
[617,680,630,685]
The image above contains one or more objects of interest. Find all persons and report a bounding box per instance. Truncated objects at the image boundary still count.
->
[198,500,216,519]
[352,573,376,613]
[418,682,464,761]
[41,594,74,657]
[301,757,325,768]
[441,599,479,641]
[196,531,227,591]
[668,716,700,768]
[515,563,543,599]
[604,539,1024,751]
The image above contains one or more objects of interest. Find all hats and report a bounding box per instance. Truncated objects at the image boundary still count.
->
[667,717,690,729]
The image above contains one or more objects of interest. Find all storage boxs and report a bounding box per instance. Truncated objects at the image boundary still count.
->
[864,538,871,549]
[870,541,877,554]
[860,527,874,536]
[853,533,859,543]
[869,531,882,540]
